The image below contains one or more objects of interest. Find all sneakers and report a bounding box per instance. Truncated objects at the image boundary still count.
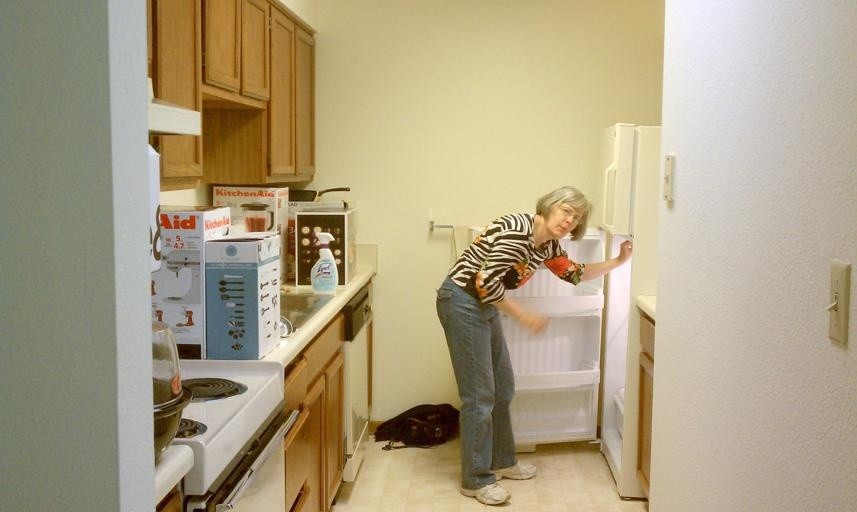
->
[491,461,537,480]
[460,483,511,505]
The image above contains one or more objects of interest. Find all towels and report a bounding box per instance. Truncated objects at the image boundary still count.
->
[451,222,470,270]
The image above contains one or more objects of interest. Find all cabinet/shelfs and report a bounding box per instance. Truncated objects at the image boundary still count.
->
[149,1,206,192]
[203,2,322,186]
[286,315,347,508]
[201,0,270,111]
[634,318,659,507]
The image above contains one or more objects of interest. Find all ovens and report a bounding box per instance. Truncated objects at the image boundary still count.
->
[187,401,300,512]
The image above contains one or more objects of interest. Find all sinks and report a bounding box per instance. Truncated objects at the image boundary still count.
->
[278,293,335,340]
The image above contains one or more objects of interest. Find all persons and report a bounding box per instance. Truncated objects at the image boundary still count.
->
[437,186,633,505]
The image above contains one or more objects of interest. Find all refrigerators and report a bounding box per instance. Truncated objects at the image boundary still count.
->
[466,123,662,500]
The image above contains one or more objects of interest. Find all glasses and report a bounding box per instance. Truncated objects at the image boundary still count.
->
[557,205,584,224]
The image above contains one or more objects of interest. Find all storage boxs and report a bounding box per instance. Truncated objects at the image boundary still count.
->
[211,184,289,286]
[207,231,283,361]
[294,206,357,288]
[151,201,231,358]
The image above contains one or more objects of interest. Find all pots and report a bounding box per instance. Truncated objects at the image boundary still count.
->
[290,188,350,202]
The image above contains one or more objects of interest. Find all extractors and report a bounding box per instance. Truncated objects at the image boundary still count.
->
[149,76,202,135]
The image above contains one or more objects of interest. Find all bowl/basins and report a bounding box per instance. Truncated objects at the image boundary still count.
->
[280,316,293,338]
[152,319,193,467]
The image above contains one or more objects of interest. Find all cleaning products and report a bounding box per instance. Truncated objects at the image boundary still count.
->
[309,232,340,293]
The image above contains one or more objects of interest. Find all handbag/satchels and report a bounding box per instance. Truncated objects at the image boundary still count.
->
[374,403,460,450]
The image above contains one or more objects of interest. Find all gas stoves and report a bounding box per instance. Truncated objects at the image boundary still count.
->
[173,359,285,496]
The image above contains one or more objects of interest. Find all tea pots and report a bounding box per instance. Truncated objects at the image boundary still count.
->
[241,204,274,232]
